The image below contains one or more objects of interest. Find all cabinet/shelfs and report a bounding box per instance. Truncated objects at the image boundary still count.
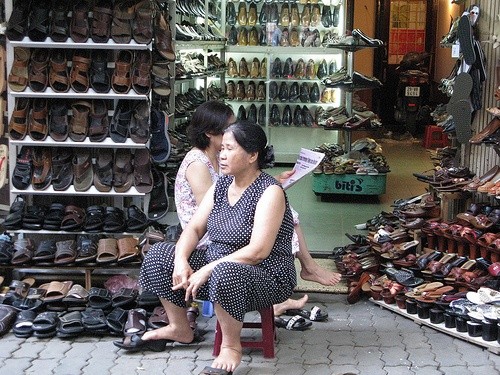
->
[368,186,500,354]
[208,0,345,164]
[0,0,227,303]
[311,44,386,201]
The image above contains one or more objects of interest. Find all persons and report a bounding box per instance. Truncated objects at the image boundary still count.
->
[174,100,341,316]
[114,122,297,375]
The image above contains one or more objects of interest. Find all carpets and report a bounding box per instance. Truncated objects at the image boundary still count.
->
[293,257,350,294]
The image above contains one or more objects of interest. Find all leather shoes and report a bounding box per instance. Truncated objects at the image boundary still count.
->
[170,0,334,125]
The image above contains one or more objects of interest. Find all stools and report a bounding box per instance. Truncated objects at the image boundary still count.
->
[423,125,449,149]
[213,305,276,358]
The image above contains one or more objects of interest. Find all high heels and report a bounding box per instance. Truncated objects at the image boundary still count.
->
[353,29,383,46]
[318,105,384,129]
[320,66,382,88]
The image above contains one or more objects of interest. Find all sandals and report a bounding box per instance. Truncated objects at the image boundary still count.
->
[307,137,391,175]
[0,0,199,334]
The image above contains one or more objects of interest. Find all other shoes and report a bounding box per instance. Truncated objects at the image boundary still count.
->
[433,7,500,155]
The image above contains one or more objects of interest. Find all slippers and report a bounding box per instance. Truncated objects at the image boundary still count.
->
[197,366,232,375]
[114,335,167,352]
[327,164,500,345]
[273,314,312,330]
[285,305,328,321]
